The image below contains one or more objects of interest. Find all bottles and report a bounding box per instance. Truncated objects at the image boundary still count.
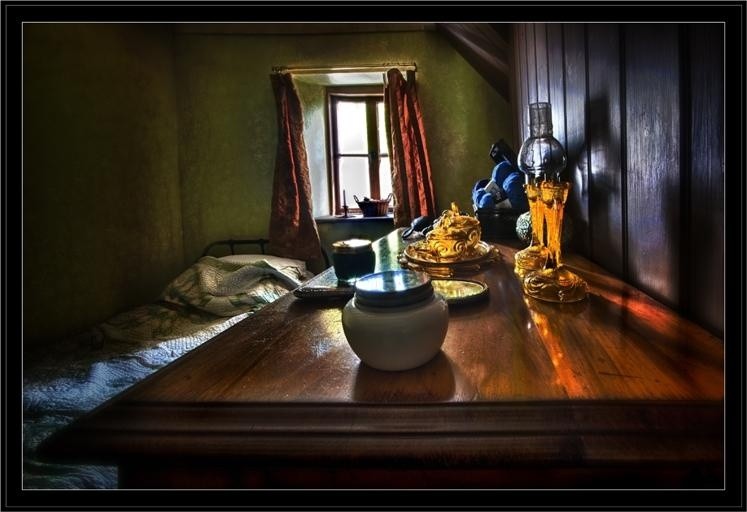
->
[333,239,375,283]
[342,269,448,371]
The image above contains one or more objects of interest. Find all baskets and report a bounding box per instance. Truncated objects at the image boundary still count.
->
[353,193,393,217]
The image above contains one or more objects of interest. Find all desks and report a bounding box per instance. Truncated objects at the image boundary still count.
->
[97,213,727,510]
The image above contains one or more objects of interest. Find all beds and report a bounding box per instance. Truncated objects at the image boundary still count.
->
[21,235,314,488]
